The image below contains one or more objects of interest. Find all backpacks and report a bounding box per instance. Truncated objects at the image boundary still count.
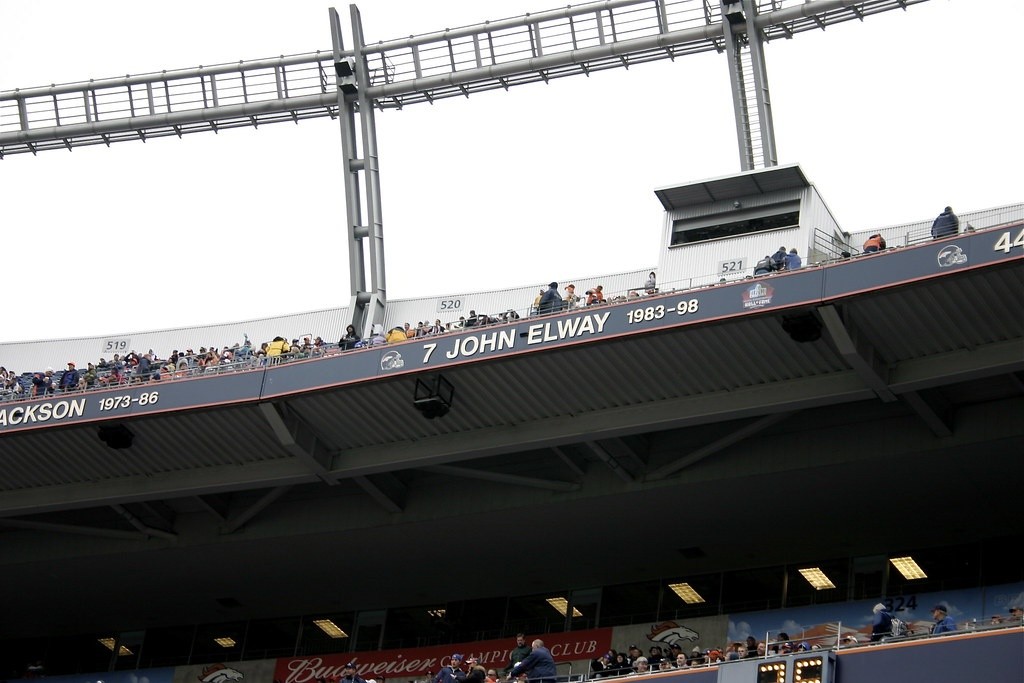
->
[880,611,907,637]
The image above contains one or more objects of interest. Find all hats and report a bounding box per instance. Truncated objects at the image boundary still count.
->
[465,658,479,663]
[452,654,462,660]
[344,663,356,669]
[692,646,699,652]
[931,605,946,612]
[782,642,793,649]
[46,367,53,372]
[637,657,648,663]
[992,615,1001,619]
[629,645,636,650]
[702,648,709,654]
[293,339,299,342]
[798,642,810,650]
[659,658,670,663]
[1009,607,1022,613]
[604,654,611,660]
[670,644,681,650]
[67,362,75,366]
[304,337,309,340]
[548,282,557,288]
[843,636,858,644]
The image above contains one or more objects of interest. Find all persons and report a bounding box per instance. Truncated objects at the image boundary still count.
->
[338,310,478,350]
[754,247,801,274]
[317,662,385,683]
[932,206,959,239]
[0,336,326,396]
[590,603,1024,678]
[426,633,556,683]
[534,271,656,314]
